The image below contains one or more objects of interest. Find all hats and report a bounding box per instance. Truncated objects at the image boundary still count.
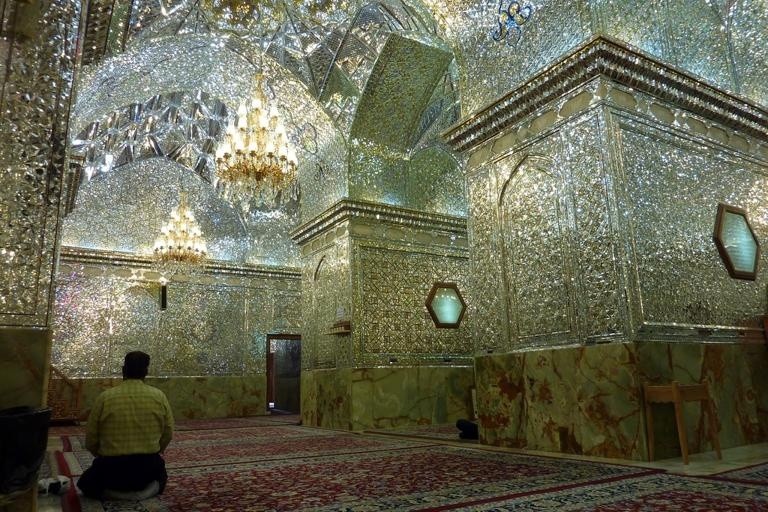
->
[124,351,151,367]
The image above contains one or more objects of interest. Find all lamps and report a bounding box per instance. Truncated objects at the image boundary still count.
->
[213,32,298,196]
[152,167,207,275]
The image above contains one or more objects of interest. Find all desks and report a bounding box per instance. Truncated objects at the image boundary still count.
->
[644,380,723,464]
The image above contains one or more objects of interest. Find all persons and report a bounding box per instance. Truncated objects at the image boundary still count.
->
[76,350,175,502]
